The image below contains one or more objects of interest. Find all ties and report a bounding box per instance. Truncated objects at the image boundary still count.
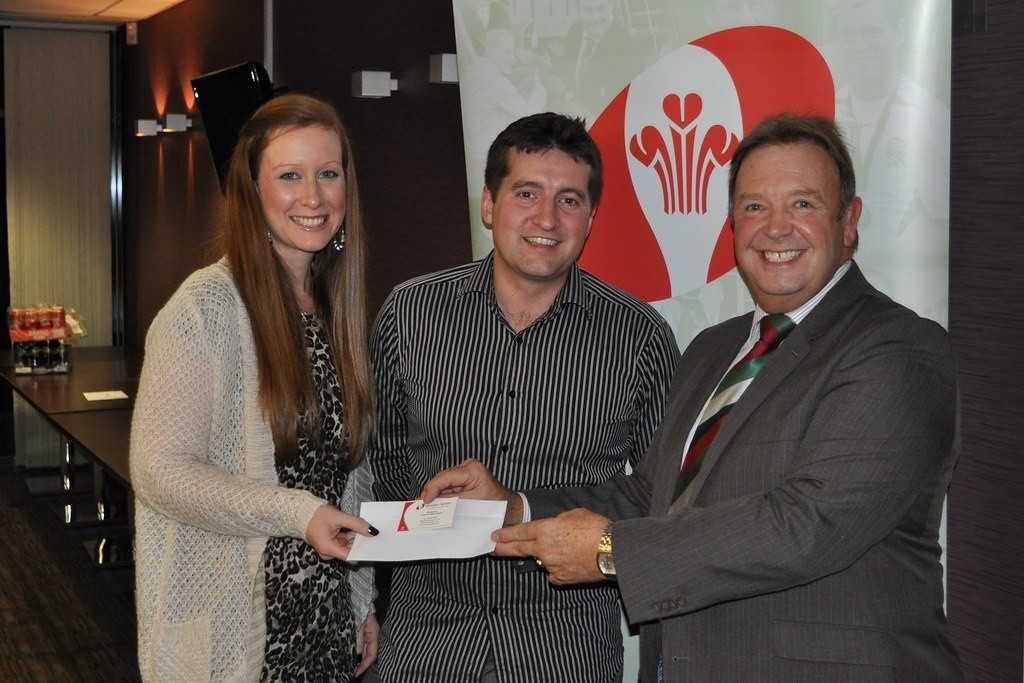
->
[666,310,794,516]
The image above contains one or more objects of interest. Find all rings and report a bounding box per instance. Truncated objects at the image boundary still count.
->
[536,559,542,567]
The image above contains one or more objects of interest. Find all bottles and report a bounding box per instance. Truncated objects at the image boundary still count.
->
[11,339,70,375]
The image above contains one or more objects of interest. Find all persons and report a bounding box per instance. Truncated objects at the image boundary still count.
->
[422,111,964,683]
[368,112,681,683]
[129,95,379,683]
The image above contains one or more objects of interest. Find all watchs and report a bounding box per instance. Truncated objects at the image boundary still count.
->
[597,522,617,578]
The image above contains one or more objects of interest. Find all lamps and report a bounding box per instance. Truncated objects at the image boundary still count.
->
[161,114,193,132]
[134,120,162,137]
[351,70,398,99]
[429,53,458,83]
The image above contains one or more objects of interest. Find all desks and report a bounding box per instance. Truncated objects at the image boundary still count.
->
[47,408,132,574]
[0,346,140,524]
